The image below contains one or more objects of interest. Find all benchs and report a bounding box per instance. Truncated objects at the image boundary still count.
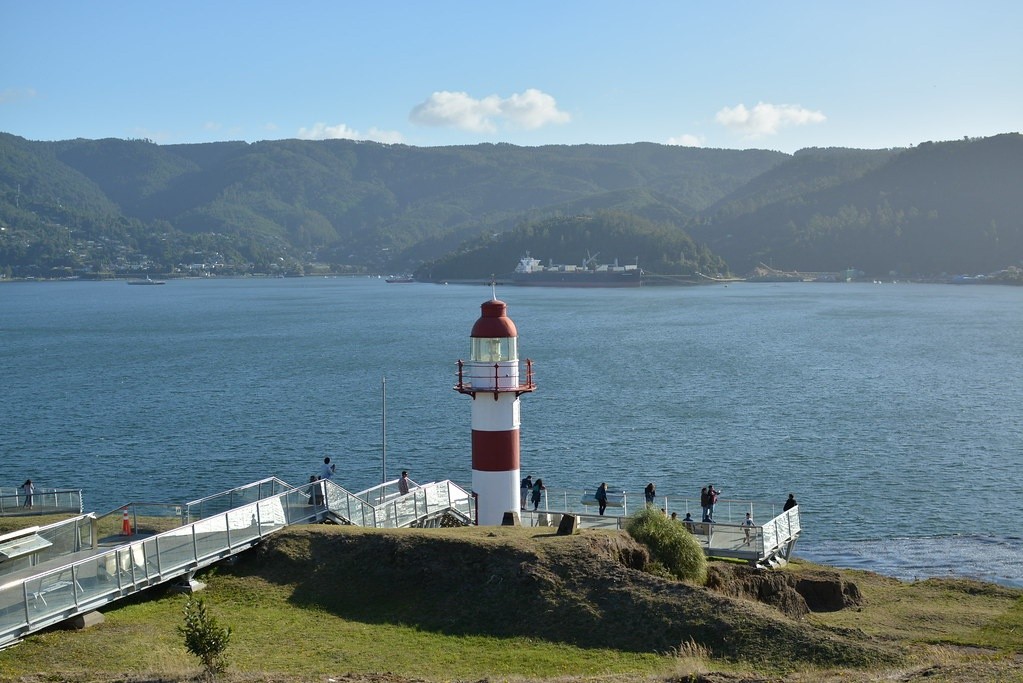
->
[618,515,634,530]
[22,564,84,612]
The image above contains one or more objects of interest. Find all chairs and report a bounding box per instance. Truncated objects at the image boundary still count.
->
[535,514,550,527]
[682,522,716,544]
[549,514,563,528]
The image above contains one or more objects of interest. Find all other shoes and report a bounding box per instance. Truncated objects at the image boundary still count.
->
[520,507,525,510]
[534,508,537,510]
[743,537,746,542]
[748,543,750,546]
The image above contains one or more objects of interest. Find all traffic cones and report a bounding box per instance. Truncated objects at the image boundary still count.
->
[121,506,133,537]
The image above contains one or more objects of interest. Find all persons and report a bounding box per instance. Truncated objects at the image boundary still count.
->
[594,483,608,516]
[531,479,546,510]
[306,457,336,522]
[740,513,756,546]
[644,483,657,510]
[22,479,35,509]
[783,494,798,511]
[398,471,410,496]
[661,485,720,533]
[519,475,533,509]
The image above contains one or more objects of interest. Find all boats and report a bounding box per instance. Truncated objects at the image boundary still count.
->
[127,275,166,285]
[385,277,415,284]
